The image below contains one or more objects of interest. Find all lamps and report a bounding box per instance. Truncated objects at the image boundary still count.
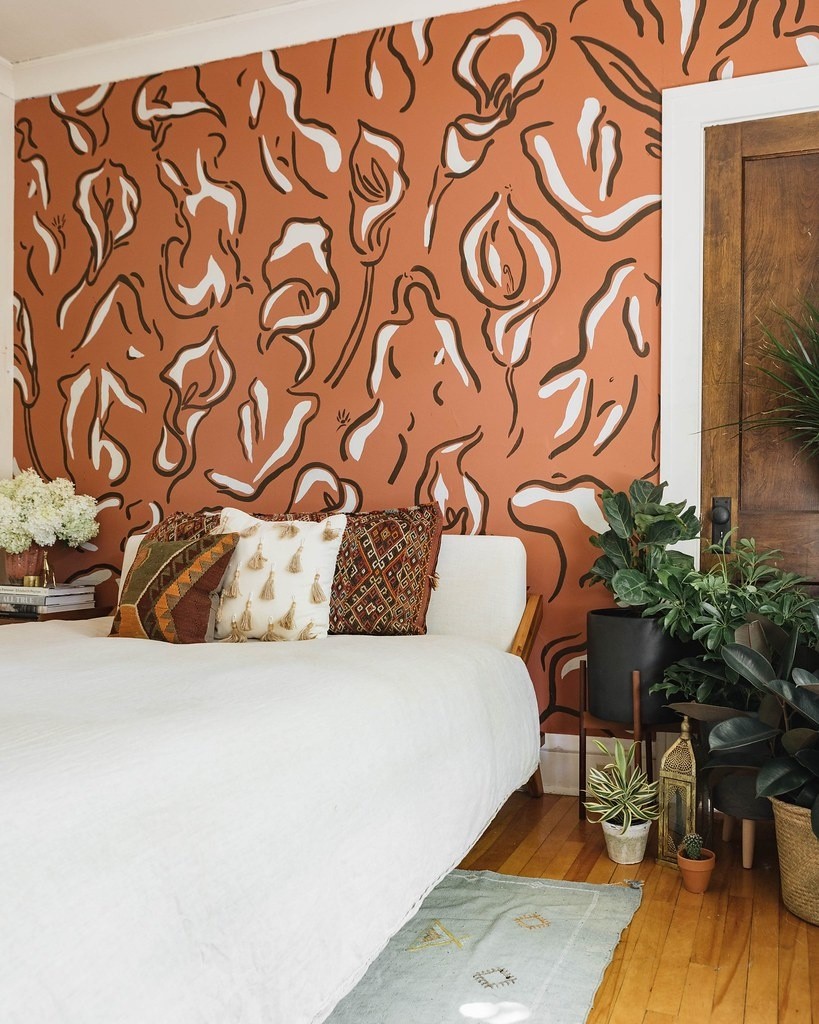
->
[654,716,713,870]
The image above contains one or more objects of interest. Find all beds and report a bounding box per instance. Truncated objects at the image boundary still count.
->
[0,535,543,1024]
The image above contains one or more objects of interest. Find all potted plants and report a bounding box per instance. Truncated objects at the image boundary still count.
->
[579,735,664,864]
[698,611,819,926]
[578,479,709,725]
[676,832,715,894]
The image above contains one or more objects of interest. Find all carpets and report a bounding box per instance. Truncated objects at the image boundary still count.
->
[326,869,643,1024]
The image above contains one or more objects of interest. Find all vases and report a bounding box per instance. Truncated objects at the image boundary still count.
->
[2,542,48,585]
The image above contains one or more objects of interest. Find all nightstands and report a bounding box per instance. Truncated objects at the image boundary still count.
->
[0,605,114,628]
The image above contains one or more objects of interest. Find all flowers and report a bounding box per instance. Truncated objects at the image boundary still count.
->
[0,467,100,554]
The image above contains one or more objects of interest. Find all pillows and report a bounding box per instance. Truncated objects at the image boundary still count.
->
[107,501,444,644]
